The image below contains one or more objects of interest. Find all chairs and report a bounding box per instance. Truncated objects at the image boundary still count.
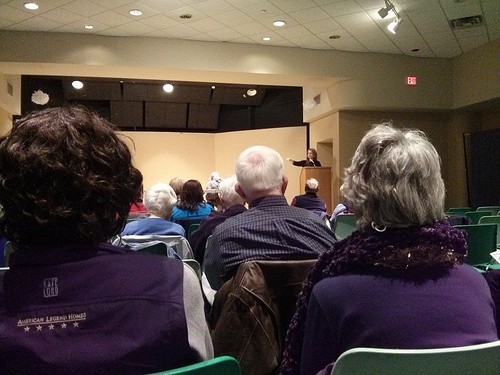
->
[113,207,500,375]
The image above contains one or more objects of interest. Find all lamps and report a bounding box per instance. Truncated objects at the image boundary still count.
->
[378,0,403,34]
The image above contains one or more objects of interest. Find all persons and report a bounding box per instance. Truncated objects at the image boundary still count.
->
[287,148,322,167]
[291,178,326,221]
[0,105,214,375]
[282,125,500,375]
[204,145,337,291]
[115,170,248,267]
